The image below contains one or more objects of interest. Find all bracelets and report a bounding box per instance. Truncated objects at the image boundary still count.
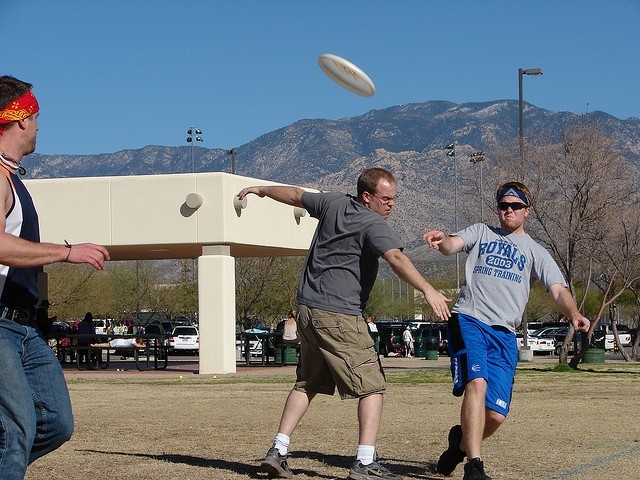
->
[63,239,72,263]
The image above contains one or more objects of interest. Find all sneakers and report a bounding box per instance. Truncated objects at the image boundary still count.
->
[347,456,405,480]
[408,353,412,358]
[438,425,467,477]
[463,458,491,480]
[262,448,292,477]
[405,354,408,358]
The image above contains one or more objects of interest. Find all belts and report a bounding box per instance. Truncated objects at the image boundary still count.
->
[0,305,34,327]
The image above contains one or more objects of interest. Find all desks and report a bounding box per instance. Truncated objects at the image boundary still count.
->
[68,332,178,371]
[237,332,273,366]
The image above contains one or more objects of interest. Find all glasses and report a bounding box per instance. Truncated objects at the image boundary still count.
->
[498,202,528,211]
[364,190,397,205]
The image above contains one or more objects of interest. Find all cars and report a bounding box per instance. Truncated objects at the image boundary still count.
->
[515,321,640,357]
[54,316,199,356]
[367,319,450,355]
[236,317,299,359]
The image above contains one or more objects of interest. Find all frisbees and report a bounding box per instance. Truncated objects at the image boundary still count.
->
[317,54,376,98]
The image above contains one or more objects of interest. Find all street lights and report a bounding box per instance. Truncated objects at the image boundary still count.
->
[518,67,545,182]
[227,148,237,175]
[186,126,203,173]
[468,151,484,223]
[445,140,460,290]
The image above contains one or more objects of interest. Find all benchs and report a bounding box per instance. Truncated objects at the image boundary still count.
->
[69,345,147,371]
[149,345,174,370]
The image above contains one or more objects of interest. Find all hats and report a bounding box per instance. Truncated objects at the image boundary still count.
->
[38,301,52,306]
[406,326,410,329]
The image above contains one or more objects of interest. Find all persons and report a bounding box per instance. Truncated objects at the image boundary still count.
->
[410,335,416,356]
[237,167,453,480]
[388,333,395,350]
[37,299,57,341]
[0,74,111,478]
[77,312,104,365]
[403,325,415,358]
[281,306,301,344]
[423,181,591,480]
[367,314,380,341]
[90,336,148,348]
